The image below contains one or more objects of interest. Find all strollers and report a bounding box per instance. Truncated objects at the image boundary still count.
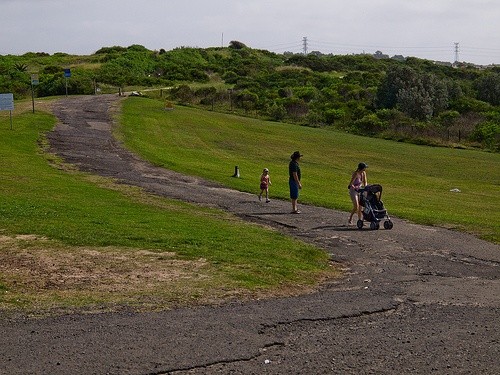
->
[354,183,393,231]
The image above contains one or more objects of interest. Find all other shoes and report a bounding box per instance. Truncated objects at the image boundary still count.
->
[259,196,262,201]
[348,216,353,226]
[266,200,270,203]
[292,209,302,214]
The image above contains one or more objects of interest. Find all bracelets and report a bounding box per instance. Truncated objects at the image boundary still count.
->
[298,185,301,186]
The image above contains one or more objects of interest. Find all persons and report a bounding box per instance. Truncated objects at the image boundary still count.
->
[348,162,367,226]
[288,151,304,215]
[258,168,272,203]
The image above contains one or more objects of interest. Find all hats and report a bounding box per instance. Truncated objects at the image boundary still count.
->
[263,168,268,172]
[291,151,303,159]
[358,163,368,168]
